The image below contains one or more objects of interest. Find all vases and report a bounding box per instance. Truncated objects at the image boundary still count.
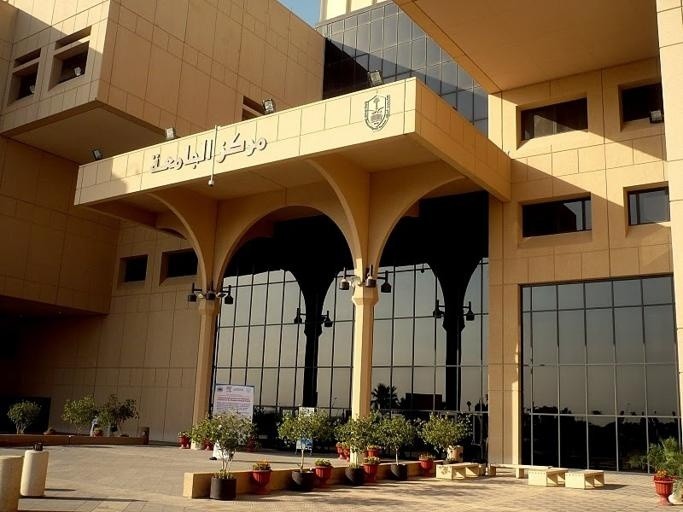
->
[654,480,675,507]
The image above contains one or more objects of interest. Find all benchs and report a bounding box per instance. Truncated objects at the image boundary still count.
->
[435,462,604,490]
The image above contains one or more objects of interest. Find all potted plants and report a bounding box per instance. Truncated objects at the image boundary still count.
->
[191,412,257,501]
[42,428,53,435]
[94,427,103,436]
[178,431,190,449]
[311,459,334,489]
[277,407,334,492]
[334,410,468,487]
[251,462,273,495]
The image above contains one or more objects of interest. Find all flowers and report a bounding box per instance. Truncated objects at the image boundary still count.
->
[654,469,672,482]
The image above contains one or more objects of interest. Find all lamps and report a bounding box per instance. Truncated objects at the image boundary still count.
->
[294,308,332,328]
[649,109,663,123]
[432,298,474,321]
[262,98,276,115]
[92,148,103,161]
[165,127,175,141]
[376,270,391,293]
[367,69,384,88]
[220,284,233,305]
[339,264,377,291]
[72,66,83,79]
[188,281,215,302]
